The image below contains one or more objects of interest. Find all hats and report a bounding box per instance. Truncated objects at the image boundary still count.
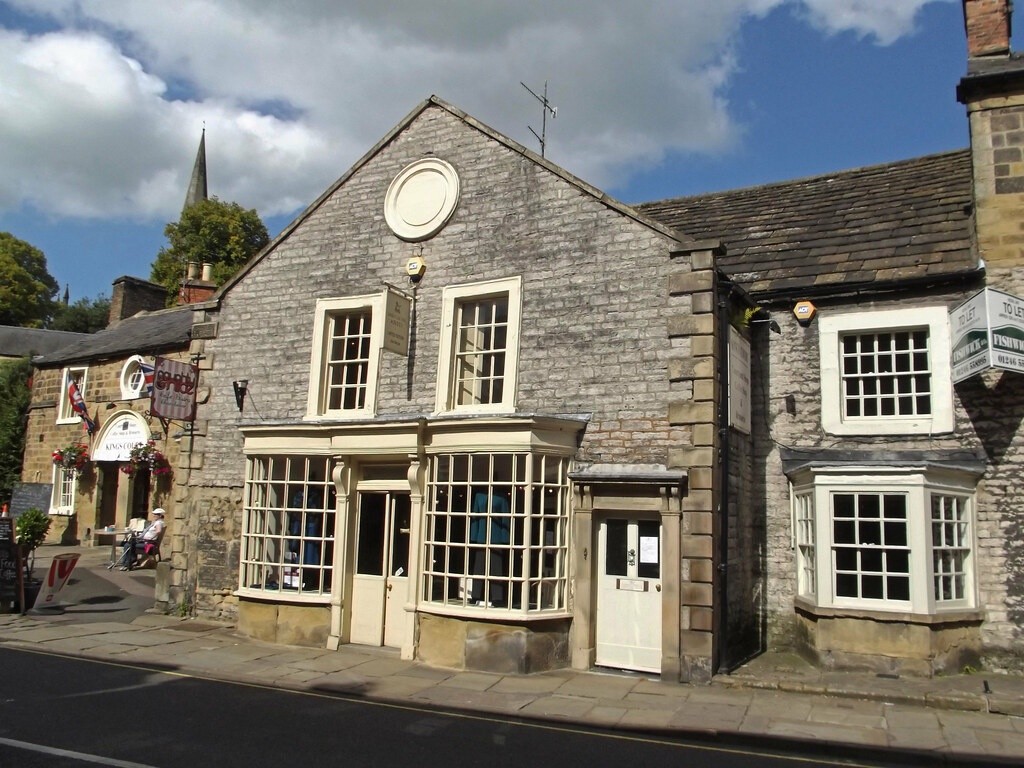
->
[153,508,166,513]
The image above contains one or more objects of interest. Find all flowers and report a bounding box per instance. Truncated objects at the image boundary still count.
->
[52,442,90,471]
[120,442,172,478]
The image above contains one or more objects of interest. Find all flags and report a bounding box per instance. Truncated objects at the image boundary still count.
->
[141,364,154,399]
[67,380,97,436]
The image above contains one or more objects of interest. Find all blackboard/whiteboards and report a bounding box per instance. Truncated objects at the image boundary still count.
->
[0,516,24,598]
[10,481,54,521]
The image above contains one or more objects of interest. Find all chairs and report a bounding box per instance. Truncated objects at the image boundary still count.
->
[129,526,167,571]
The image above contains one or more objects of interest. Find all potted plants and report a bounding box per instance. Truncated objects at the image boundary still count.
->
[14,507,53,612]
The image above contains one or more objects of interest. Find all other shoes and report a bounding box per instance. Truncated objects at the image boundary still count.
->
[119,565,127,571]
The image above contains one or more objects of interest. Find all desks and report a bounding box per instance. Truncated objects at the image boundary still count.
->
[91,528,144,567]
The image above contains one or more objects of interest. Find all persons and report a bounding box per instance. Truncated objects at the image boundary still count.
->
[118,508,165,572]
